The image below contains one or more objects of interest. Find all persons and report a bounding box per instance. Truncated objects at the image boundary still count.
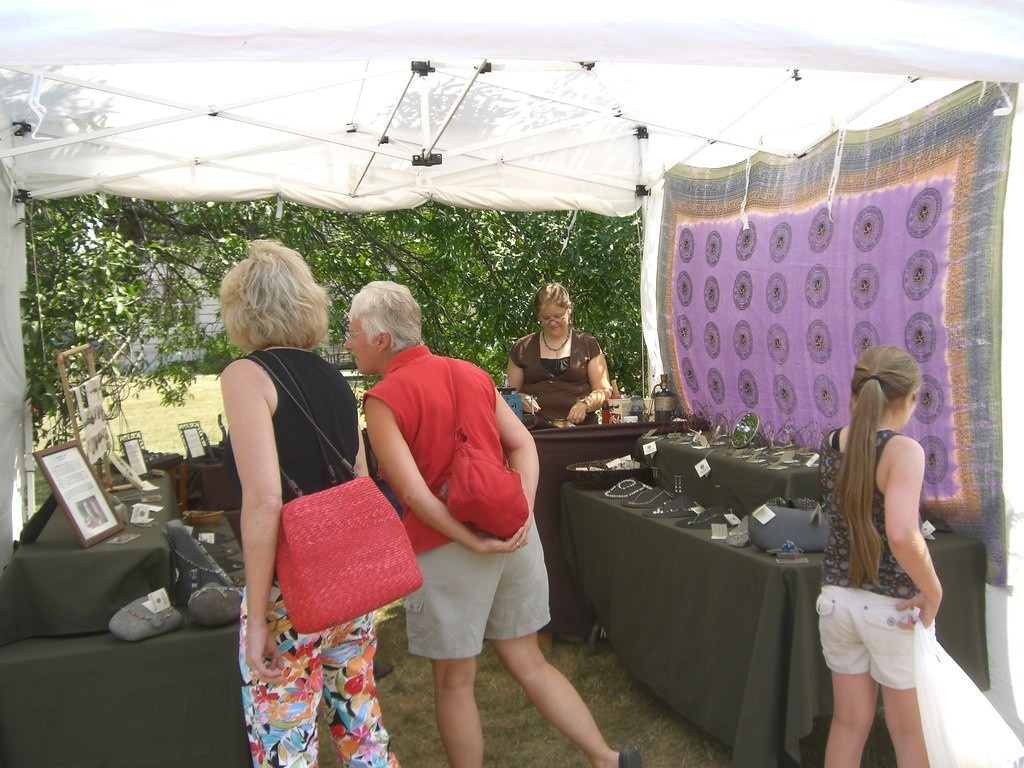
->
[344,280,642,768]
[816,344,942,768]
[218,239,402,768]
[507,283,612,427]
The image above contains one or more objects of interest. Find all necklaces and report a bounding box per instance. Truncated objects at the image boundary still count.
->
[606,426,815,552]
[542,326,570,351]
[120,483,243,629]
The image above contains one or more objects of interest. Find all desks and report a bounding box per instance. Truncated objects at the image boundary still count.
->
[558,433,992,768]
[180,442,242,547]
[531,420,708,647]
[0,469,252,768]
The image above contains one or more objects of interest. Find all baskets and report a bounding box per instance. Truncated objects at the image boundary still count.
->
[566,458,653,489]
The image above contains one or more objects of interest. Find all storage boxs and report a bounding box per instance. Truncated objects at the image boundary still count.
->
[602,399,654,425]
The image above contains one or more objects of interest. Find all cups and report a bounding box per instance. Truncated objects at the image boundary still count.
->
[630,411,643,422]
[624,416,638,422]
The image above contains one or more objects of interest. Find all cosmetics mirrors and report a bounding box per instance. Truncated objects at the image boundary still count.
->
[731,413,760,448]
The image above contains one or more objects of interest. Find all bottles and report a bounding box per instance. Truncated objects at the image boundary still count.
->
[631,396,644,410]
[602,400,610,424]
[655,374,675,422]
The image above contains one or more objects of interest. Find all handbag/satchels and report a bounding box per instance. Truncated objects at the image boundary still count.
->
[446,442,530,539]
[910,607,1024,768]
[275,475,424,635]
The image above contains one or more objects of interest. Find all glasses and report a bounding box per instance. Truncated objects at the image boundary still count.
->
[538,310,567,325]
[342,330,362,340]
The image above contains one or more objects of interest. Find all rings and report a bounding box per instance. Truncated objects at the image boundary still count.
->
[574,413,577,415]
[250,670,259,676]
[515,541,522,549]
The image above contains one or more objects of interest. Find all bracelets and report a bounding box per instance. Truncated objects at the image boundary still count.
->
[580,399,591,414]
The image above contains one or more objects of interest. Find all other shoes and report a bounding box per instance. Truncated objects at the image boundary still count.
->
[619,746,641,768]
